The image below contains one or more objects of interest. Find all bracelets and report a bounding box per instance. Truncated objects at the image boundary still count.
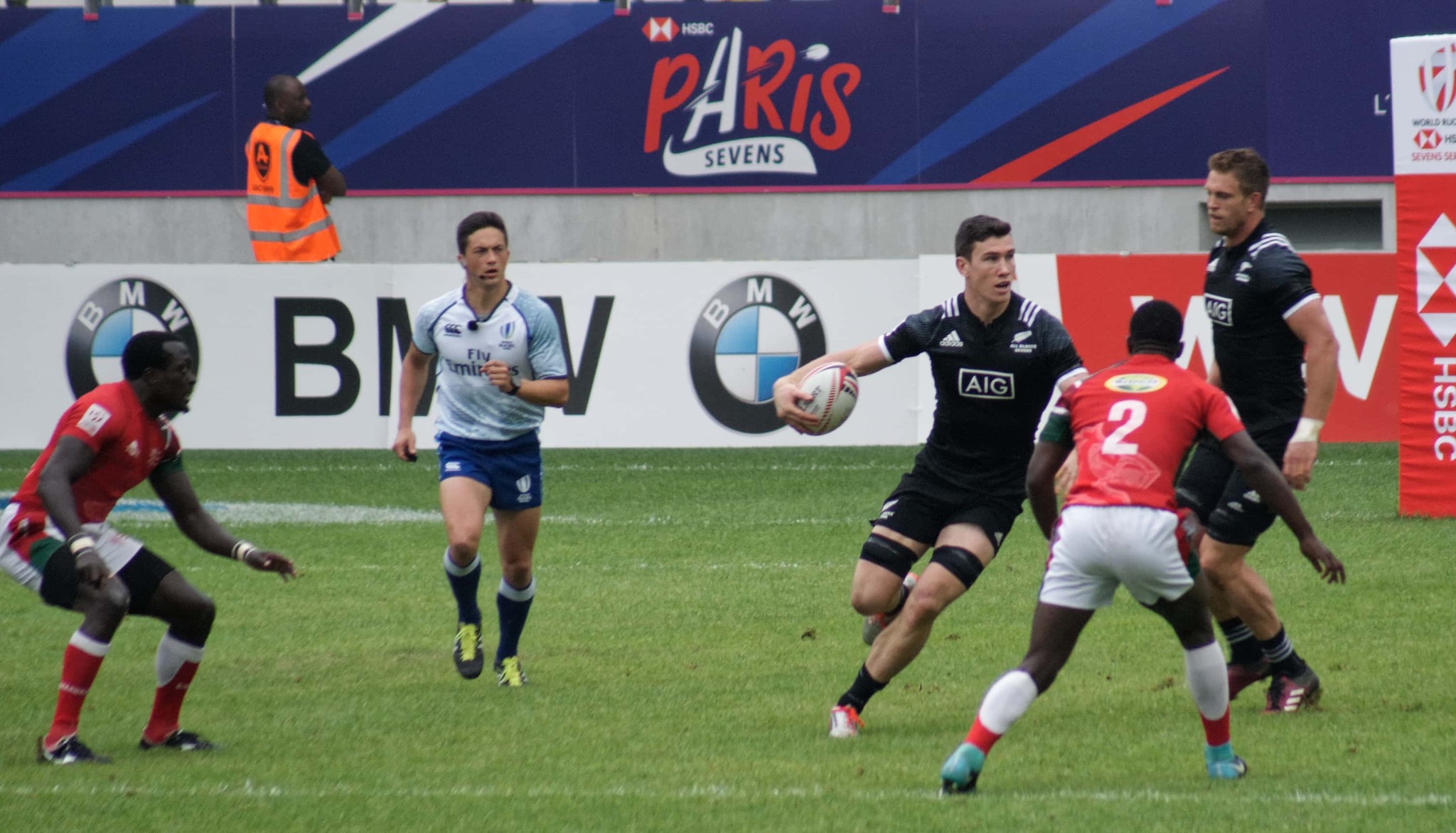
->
[1291,418,1325,443]
[231,540,257,561]
[67,532,97,559]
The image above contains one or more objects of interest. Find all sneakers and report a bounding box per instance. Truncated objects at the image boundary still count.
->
[827,705,861,738]
[453,610,484,680]
[493,656,525,687]
[1209,754,1246,784]
[37,737,106,765]
[1228,653,1264,697]
[137,731,211,752]
[862,572,920,646]
[937,773,976,799]
[1264,666,1318,714]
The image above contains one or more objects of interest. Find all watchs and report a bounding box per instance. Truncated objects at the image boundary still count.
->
[506,375,521,396]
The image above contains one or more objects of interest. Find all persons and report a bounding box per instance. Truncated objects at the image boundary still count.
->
[393,216,570,688]
[773,215,1091,739]
[0,337,298,768]
[939,298,1346,793]
[1174,146,1340,712]
[244,74,346,263]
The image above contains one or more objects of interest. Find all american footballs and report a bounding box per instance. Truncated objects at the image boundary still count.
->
[795,361,860,437]
